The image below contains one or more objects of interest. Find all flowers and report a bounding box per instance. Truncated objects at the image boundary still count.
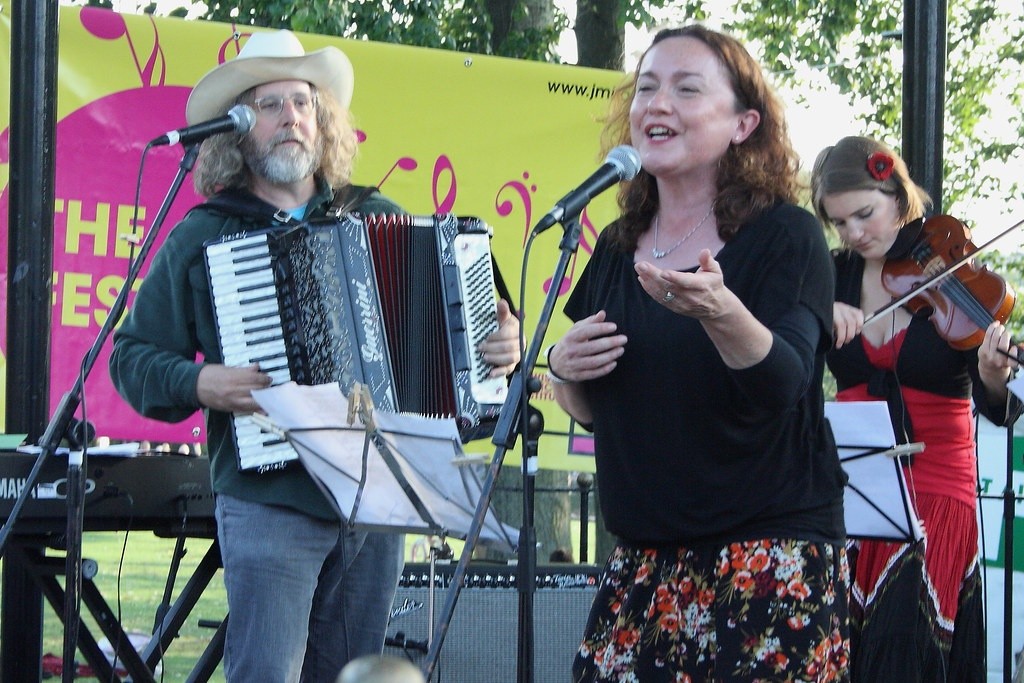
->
[867,152,895,181]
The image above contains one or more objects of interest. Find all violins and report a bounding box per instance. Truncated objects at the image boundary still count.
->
[881,214,1024,366]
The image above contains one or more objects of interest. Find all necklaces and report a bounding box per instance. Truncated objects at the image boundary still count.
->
[652,208,713,258]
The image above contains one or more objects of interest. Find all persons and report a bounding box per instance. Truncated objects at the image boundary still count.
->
[810,135,1024,683]
[543,24,852,683]
[105,30,527,683]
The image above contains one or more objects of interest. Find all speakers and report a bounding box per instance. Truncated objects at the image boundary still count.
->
[390,563,604,683]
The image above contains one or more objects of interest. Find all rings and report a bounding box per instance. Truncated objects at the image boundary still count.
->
[662,292,675,303]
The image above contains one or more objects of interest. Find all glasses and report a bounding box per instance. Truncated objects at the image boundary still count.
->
[255,89,318,118]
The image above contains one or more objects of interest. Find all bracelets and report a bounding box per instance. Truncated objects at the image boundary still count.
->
[544,344,571,383]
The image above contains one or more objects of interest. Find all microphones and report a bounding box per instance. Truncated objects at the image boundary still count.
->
[152,104,256,147]
[532,145,643,234]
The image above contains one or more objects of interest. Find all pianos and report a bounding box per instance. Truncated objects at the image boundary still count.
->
[0,447,231,683]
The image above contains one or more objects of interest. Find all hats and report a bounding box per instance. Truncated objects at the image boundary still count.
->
[185,28,355,127]
